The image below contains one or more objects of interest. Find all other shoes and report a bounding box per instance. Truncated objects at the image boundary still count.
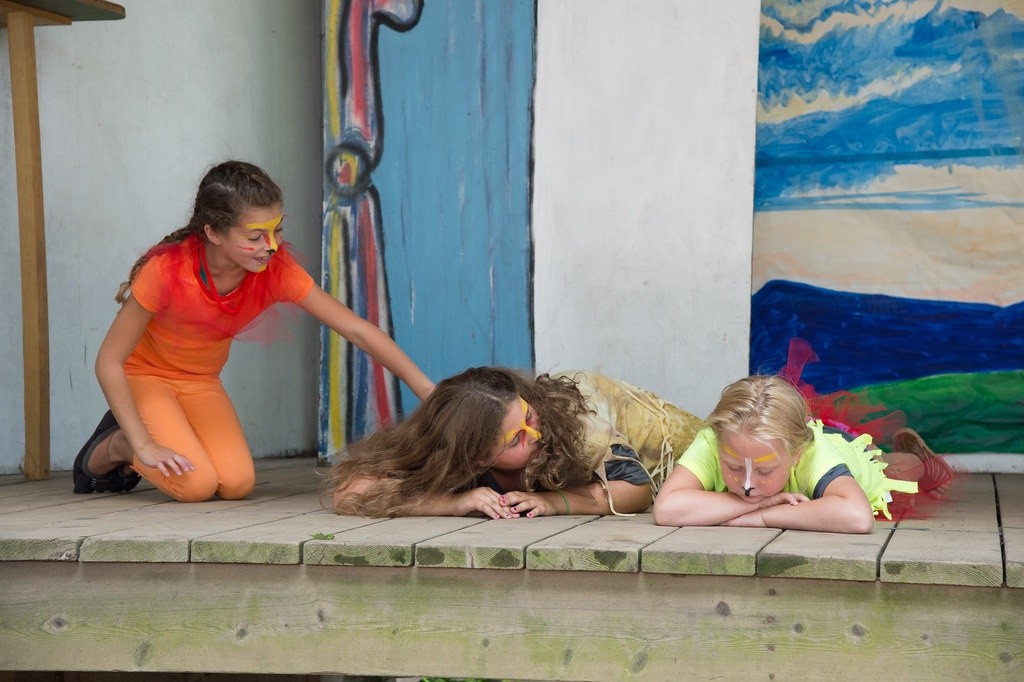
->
[72,408,141,495]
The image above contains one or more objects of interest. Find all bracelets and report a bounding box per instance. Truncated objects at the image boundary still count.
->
[556,490,569,514]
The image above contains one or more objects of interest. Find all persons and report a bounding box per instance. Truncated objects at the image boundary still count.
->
[73,161,436,503]
[329,367,705,519]
[654,374,951,534]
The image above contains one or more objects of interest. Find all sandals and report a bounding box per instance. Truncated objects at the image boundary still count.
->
[892,427,954,500]
